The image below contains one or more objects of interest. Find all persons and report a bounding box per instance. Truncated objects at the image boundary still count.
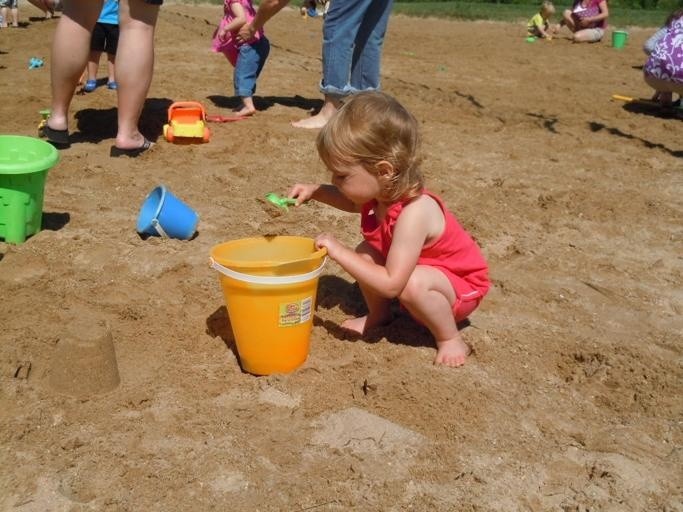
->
[236,0,391,129]
[205,0,270,116]
[287,90,489,368]
[562,0,609,43]
[84,0,120,92]
[47,0,163,151]
[643,9,683,109]
[527,0,555,42]
[0,0,19,27]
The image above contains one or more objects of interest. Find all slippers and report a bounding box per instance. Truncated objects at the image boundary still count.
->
[43,123,69,143]
[110,136,151,158]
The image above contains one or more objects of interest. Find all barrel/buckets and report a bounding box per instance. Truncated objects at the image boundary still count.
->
[612,31,628,48]
[207,235,328,376]
[136,185,199,241]
[0,134,59,244]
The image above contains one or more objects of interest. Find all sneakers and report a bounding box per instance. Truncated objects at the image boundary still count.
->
[106,81,118,89]
[84,80,97,92]
